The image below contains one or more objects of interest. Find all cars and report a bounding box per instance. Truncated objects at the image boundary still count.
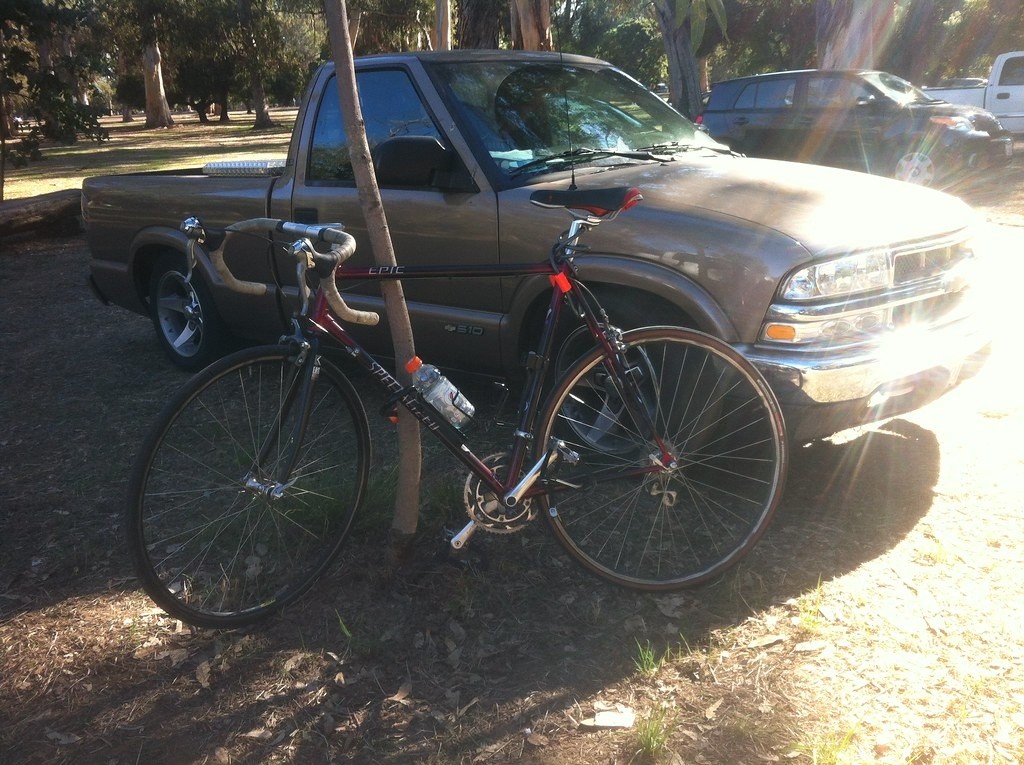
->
[692,50,1024,188]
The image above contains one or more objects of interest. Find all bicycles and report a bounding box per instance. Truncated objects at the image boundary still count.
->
[126,184,789,629]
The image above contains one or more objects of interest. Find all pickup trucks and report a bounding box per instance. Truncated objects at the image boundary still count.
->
[81,48,988,471]
